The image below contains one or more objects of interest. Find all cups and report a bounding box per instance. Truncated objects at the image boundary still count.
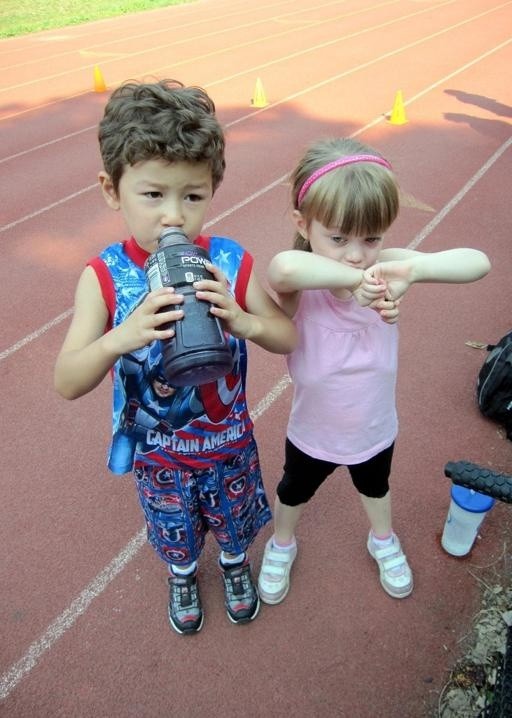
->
[439,482,495,558]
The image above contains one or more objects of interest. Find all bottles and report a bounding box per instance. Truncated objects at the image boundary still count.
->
[142,226,234,388]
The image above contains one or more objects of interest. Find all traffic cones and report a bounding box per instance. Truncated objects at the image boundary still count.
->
[386,87,409,126]
[249,74,268,110]
[90,63,112,95]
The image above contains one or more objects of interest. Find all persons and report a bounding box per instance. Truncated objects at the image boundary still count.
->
[257,135,492,606]
[51,81,300,638]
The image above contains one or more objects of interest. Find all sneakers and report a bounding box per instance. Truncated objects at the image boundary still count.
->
[219,536,297,624]
[367,530,414,599]
[168,564,204,635]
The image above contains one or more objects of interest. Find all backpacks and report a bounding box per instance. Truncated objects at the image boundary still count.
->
[477,332,512,440]
